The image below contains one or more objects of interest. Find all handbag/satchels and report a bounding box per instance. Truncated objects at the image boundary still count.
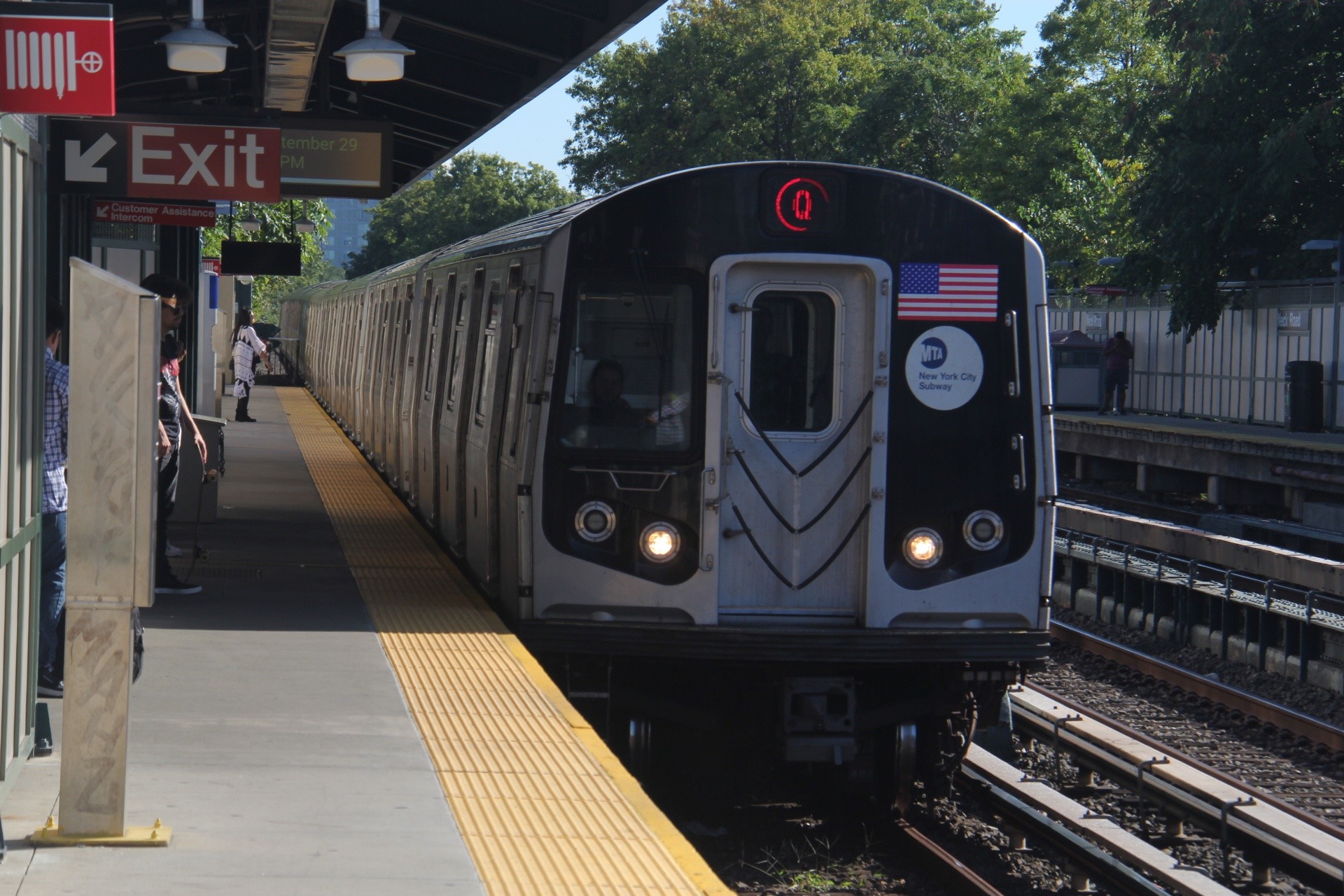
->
[229,359,234,371]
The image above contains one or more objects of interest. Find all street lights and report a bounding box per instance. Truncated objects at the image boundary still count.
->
[1299,235,1344,432]
[1098,256,1132,336]
[1052,259,1075,332]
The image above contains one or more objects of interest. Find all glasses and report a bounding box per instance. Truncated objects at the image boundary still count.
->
[162,302,186,315]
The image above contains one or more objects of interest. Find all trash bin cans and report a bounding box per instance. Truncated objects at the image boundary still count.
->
[1052,330,1119,417]
[1285,361,1323,433]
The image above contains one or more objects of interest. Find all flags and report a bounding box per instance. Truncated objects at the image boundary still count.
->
[898,260,999,321]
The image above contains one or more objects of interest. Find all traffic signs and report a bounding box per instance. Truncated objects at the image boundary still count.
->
[94,200,218,229]
[44,115,286,205]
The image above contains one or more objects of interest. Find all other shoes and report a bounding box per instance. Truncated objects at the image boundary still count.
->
[235,415,256,422]
[154,567,202,594]
[38,667,64,696]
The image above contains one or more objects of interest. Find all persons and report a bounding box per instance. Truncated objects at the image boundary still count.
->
[227,308,273,423]
[1098,331,1133,416]
[140,273,207,595]
[36,304,69,697]
[564,360,658,448]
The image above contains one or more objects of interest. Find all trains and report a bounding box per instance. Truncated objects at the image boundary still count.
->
[279,159,1061,820]
